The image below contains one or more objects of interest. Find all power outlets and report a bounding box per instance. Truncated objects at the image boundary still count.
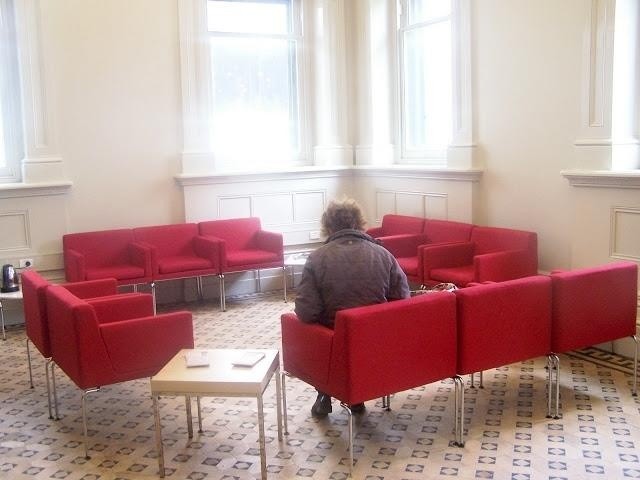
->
[19,259,34,268]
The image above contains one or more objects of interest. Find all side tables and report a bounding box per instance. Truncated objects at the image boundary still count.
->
[285,252,311,289]
[150,348,281,480]
[0,281,24,340]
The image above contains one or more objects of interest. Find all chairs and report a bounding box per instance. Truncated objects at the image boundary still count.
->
[46,283,195,461]
[454,275,554,447]
[423,225,539,289]
[133,223,223,317]
[20,270,142,419]
[280,290,460,477]
[365,214,430,241]
[198,217,287,312]
[482,261,640,419]
[376,218,478,285]
[62,228,153,293]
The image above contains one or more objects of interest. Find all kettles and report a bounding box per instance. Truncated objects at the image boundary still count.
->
[1,264,20,292]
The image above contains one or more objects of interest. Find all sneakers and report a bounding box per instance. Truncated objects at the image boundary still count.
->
[311,390,332,415]
[339,400,366,412]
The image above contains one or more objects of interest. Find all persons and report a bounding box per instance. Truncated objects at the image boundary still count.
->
[294,198,411,415]
